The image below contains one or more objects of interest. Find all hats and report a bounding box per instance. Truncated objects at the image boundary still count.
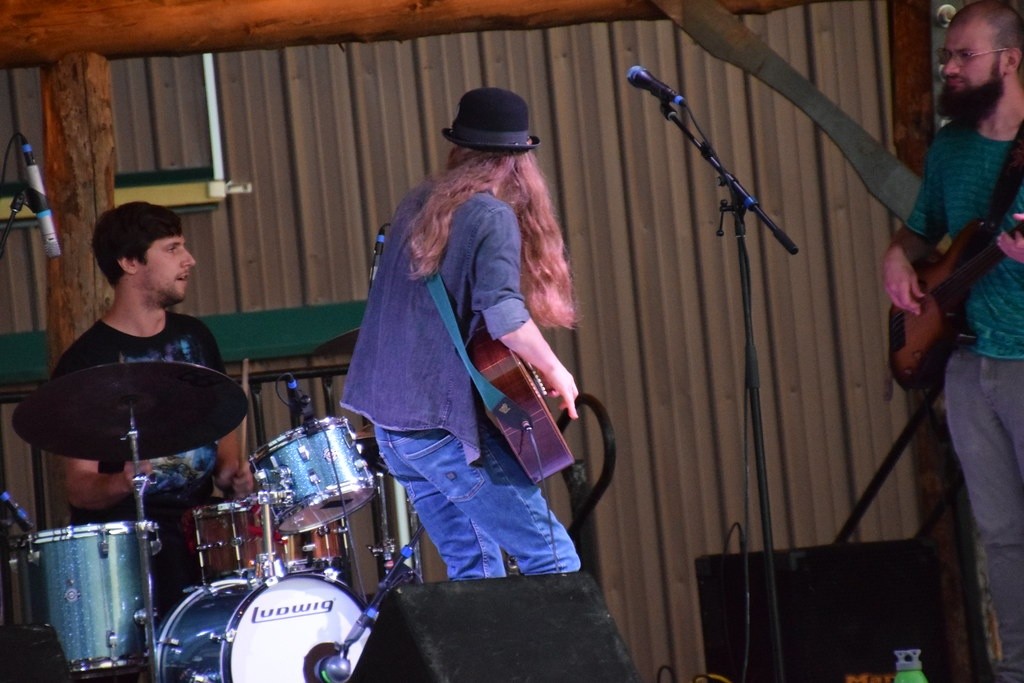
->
[442,87,541,149]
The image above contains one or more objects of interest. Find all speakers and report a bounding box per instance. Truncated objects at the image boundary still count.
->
[345,570,646,683]
[696,539,955,683]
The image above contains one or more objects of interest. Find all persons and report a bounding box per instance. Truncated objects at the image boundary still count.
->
[879,2,1023,682]
[343,86,583,578]
[49,202,255,607]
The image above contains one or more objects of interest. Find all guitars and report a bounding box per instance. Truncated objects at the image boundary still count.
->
[887,217,1023,391]
[468,325,578,487]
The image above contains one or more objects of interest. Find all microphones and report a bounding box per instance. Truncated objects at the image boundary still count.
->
[936,4,957,28]
[626,66,687,107]
[286,374,301,412]
[0,489,34,531]
[314,650,352,683]
[21,135,61,258]
[369,230,386,291]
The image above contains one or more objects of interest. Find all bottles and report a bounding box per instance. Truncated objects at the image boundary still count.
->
[894,649,928,683]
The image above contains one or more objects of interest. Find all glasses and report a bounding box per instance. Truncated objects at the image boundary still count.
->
[936,47,1010,64]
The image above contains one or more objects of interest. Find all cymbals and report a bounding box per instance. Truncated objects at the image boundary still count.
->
[12,357,251,463]
[308,324,365,366]
[355,424,377,440]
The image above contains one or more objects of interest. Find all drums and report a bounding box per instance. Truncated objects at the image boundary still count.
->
[11,518,154,678]
[191,497,290,582]
[282,515,348,569]
[250,415,381,535]
[149,571,376,683]
